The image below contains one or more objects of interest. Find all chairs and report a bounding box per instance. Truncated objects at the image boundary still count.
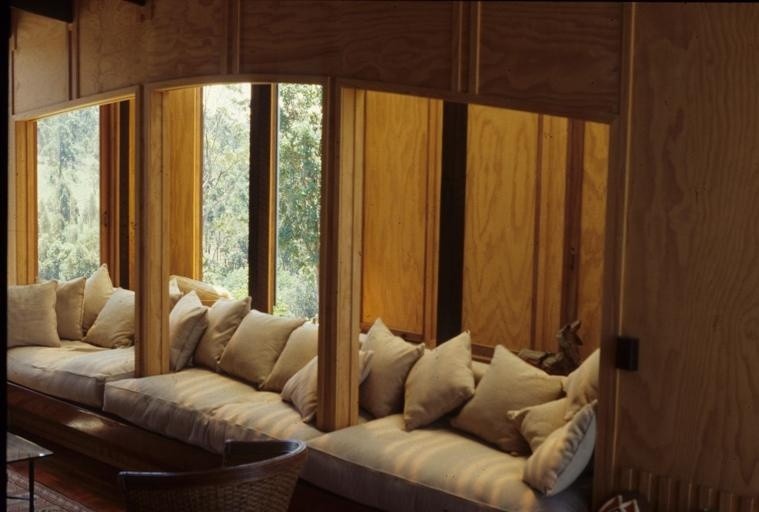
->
[117,438,309,512]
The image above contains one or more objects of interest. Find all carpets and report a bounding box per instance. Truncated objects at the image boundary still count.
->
[7,463,95,512]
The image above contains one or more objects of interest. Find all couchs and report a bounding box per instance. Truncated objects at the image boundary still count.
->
[7,322,608,511]
